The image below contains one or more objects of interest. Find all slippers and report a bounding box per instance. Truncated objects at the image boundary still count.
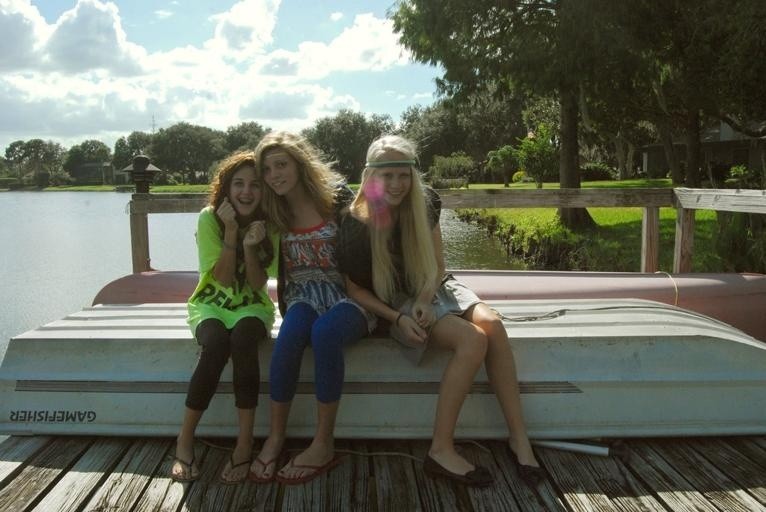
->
[276,451,343,486]
[221,452,251,486]
[171,446,201,482]
[248,445,283,484]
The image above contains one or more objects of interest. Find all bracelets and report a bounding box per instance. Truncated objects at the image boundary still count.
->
[395,311,405,328]
[221,240,237,251]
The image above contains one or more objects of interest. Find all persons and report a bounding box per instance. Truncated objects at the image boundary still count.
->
[246,129,385,486]
[170,151,283,487]
[333,134,545,490]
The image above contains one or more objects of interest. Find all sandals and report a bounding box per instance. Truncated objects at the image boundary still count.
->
[422,451,494,486]
[505,442,546,480]
